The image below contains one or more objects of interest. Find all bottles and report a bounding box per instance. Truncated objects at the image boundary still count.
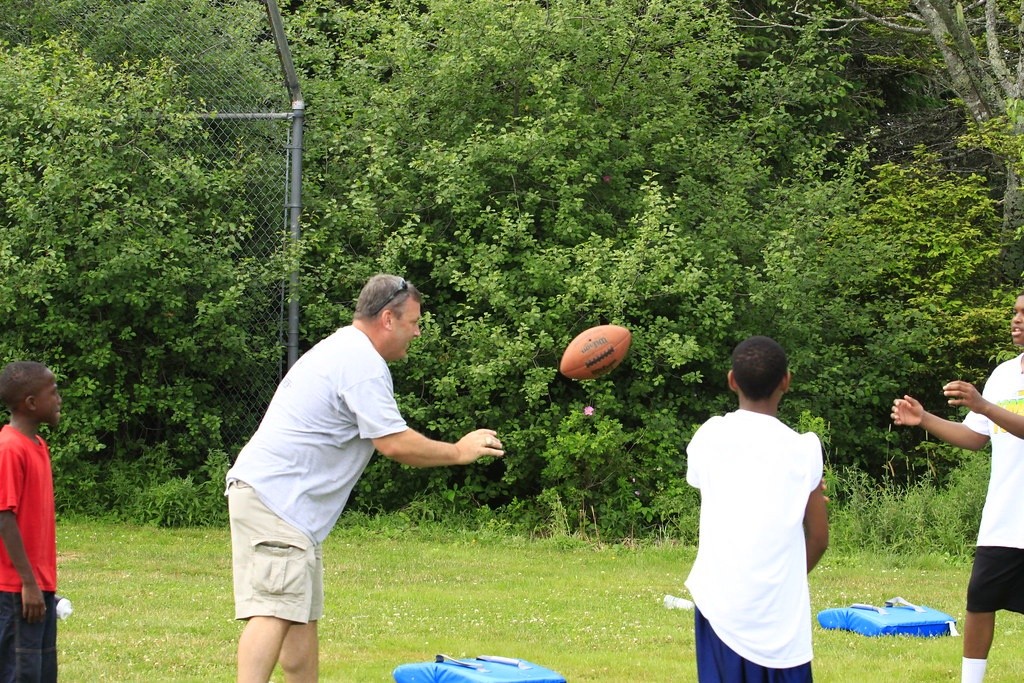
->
[55,594,74,621]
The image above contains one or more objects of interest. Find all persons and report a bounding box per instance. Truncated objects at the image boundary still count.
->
[0,361,61,683]
[891,289,1024,683]
[687,335,828,683]
[223,274,504,682]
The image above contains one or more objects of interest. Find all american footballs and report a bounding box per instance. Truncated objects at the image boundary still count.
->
[557,323,631,379]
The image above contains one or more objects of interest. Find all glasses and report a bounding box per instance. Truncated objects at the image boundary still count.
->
[367,277,408,318]
